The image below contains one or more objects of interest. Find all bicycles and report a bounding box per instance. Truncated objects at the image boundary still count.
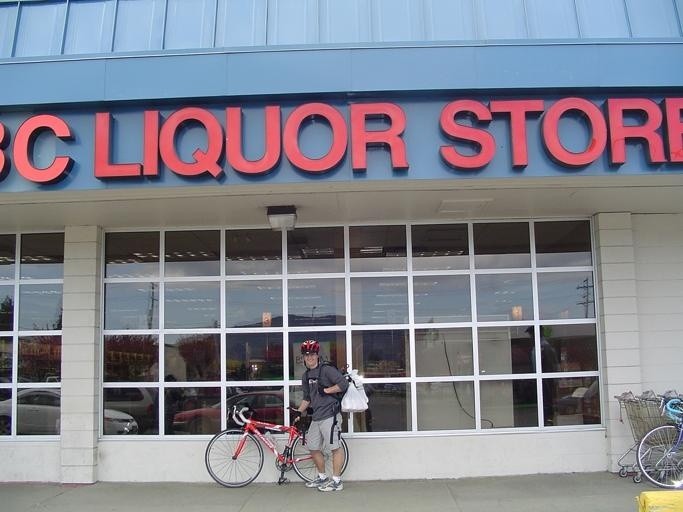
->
[202,401,350,488]
[636,391,683,489]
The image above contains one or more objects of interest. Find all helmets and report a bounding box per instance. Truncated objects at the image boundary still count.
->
[301,340,320,355]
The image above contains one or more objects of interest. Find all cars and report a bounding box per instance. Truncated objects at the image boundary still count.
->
[171,390,296,434]
[0,389,155,435]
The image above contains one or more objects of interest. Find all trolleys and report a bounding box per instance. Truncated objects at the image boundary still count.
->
[614,388,683,484]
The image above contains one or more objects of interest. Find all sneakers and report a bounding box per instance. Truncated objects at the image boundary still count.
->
[306,475,344,492]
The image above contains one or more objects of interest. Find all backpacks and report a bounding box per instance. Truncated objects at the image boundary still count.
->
[318,361,368,412]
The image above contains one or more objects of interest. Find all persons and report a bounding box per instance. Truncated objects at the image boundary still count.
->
[524,325,559,426]
[292,339,349,492]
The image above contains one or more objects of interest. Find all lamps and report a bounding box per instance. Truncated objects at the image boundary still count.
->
[266,205,298,232]
